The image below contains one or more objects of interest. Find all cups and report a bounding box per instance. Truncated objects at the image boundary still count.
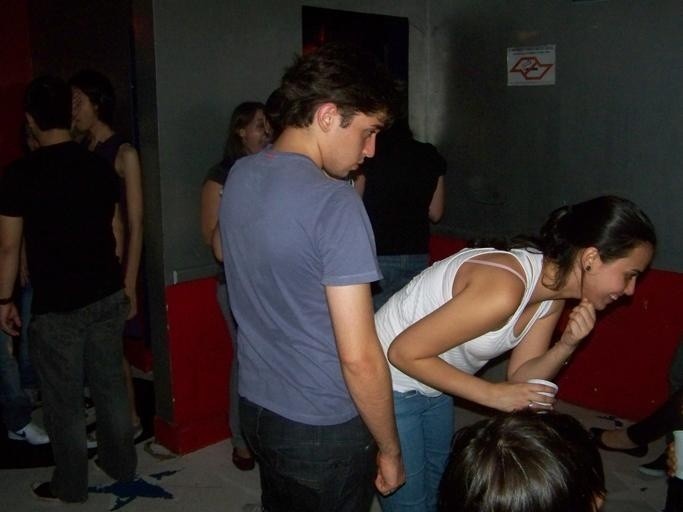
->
[527,378,558,414]
[673,430,682,478]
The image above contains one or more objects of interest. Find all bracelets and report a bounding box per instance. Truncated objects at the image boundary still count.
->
[0,297,13,308]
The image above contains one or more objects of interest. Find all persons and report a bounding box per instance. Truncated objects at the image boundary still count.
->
[0,327,51,448]
[0,72,140,507]
[67,68,146,451]
[369,191,655,509]
[580,380,681,511]
[432,405,607,512]
[196,98,276,470]
[15,117,43,401]
[216,37,406,510]
[351,118,445,314]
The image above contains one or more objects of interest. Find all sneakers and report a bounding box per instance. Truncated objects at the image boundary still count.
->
[82,398,143,449]
[30,480,58,501]
[22,387,41,409]
[232,447,255,470]
[638,453,666,476]
[588,427,648,456]
[9,421,50,444]
